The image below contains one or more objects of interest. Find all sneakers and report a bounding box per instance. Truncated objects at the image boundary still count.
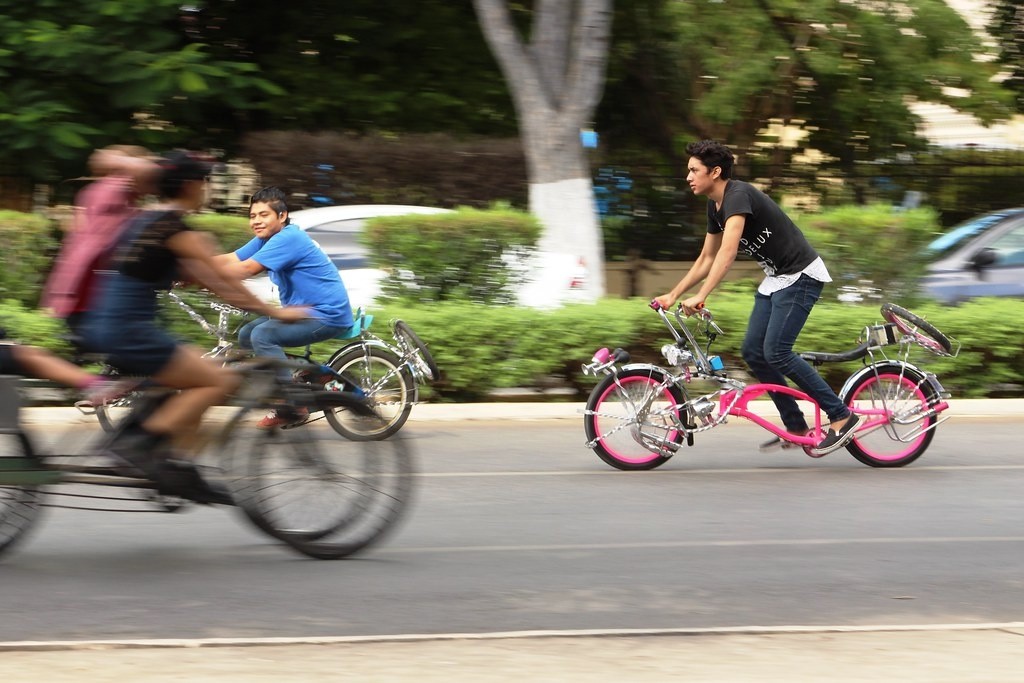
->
[256,402,309,430]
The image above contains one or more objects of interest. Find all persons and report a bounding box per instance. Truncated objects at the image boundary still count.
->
[76,159,312,479]
[34,144,162,373]
[0,326,112,390]
[652,139,863,453]
[210,185,352,430]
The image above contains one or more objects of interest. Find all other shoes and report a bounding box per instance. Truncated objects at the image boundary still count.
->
[758,426,813,453]
[95,426,226,514]
[816,410,862,456]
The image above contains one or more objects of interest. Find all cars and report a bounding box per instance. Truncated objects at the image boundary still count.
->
[239,205,594,310]
[832,207,1023,306]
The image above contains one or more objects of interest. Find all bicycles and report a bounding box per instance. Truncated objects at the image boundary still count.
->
[0,317,412,560]
[93,280,442,442]
[581,299,961,472]
